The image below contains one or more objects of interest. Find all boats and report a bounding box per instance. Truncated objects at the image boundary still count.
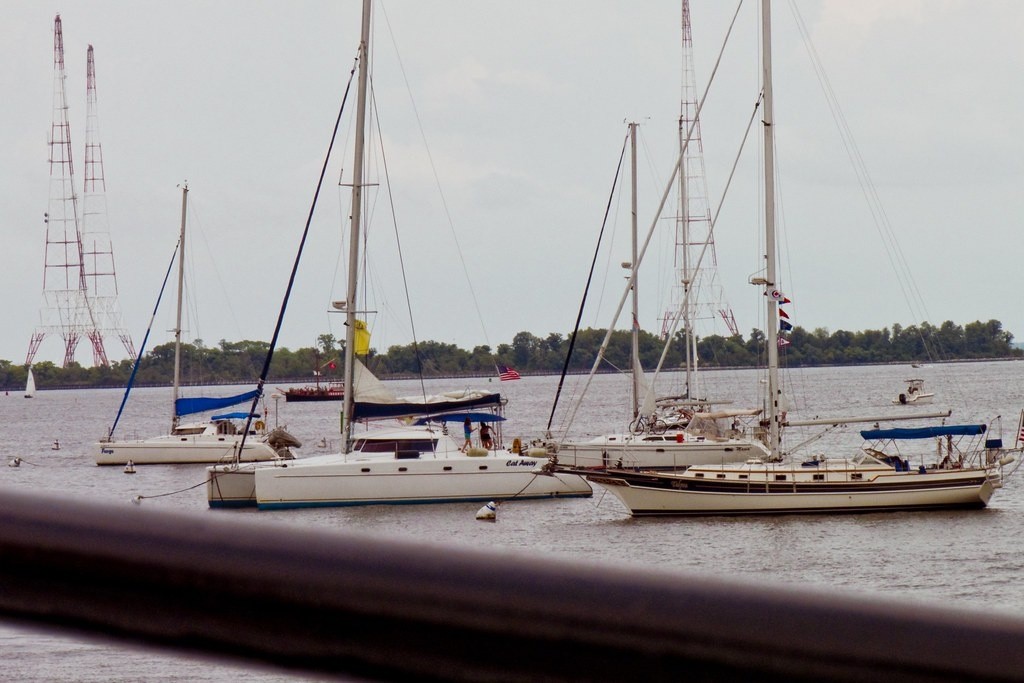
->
[891,374,935,406]
[274,338,345,403]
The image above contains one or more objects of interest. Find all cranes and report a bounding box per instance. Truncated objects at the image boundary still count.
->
[62,41,140,370]
[24,9,111,373]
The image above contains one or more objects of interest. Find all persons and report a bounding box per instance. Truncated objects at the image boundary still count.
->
[399,416,415,424]
[479,421,496,450]
[461,418,478,453]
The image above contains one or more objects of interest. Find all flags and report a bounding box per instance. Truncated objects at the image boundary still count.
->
[1019,416,1024,440]
[498,366,521,381]
[764,288,792,331]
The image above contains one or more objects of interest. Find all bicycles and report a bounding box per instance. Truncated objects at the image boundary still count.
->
[629,410,668,436]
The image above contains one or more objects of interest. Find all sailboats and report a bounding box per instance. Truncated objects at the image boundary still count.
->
[23,366,36,399]
[92,0,1024,519]
